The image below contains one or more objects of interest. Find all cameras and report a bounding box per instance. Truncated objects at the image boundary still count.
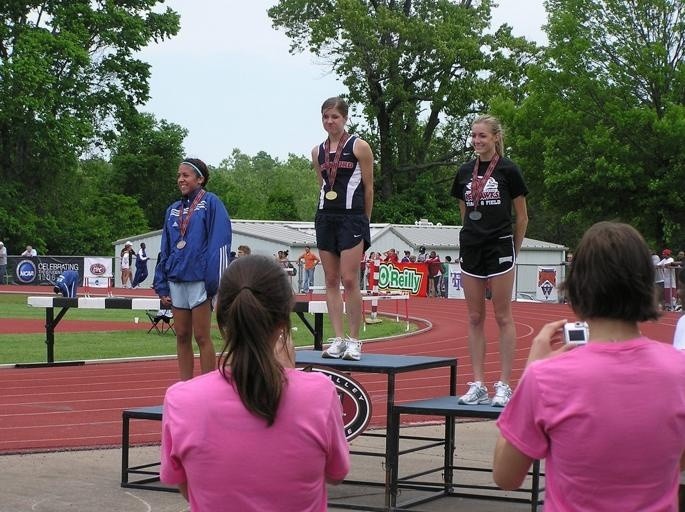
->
[562,321,590,346]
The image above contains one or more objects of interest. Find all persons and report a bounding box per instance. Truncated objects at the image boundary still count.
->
[450,114,529,408]
[158,254,349,512]
[0,241,8,285]
[492,221,684,512]
[359,245,463,299]
[121,241,150,290]
[310,95,375,361]
[229,245,320,295]
[54,269,79,298]
[153,156,232,381]
[21,245,37,258]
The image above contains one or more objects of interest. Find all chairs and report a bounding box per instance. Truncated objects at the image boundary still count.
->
[145,300,174,337]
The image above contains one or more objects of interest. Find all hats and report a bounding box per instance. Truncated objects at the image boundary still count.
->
[662,249,670,255]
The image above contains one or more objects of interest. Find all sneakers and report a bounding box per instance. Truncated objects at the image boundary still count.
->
[491,381,512,407]
[458,381,488,405]
[342,333,362,361]
[321,337,347,359]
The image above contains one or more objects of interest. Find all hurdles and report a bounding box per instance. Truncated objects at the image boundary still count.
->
[0,296,346,368]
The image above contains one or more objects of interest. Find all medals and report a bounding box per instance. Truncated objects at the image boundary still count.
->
[176,240,187,249]
[468,210,483,221]
[325,190,338,200]
